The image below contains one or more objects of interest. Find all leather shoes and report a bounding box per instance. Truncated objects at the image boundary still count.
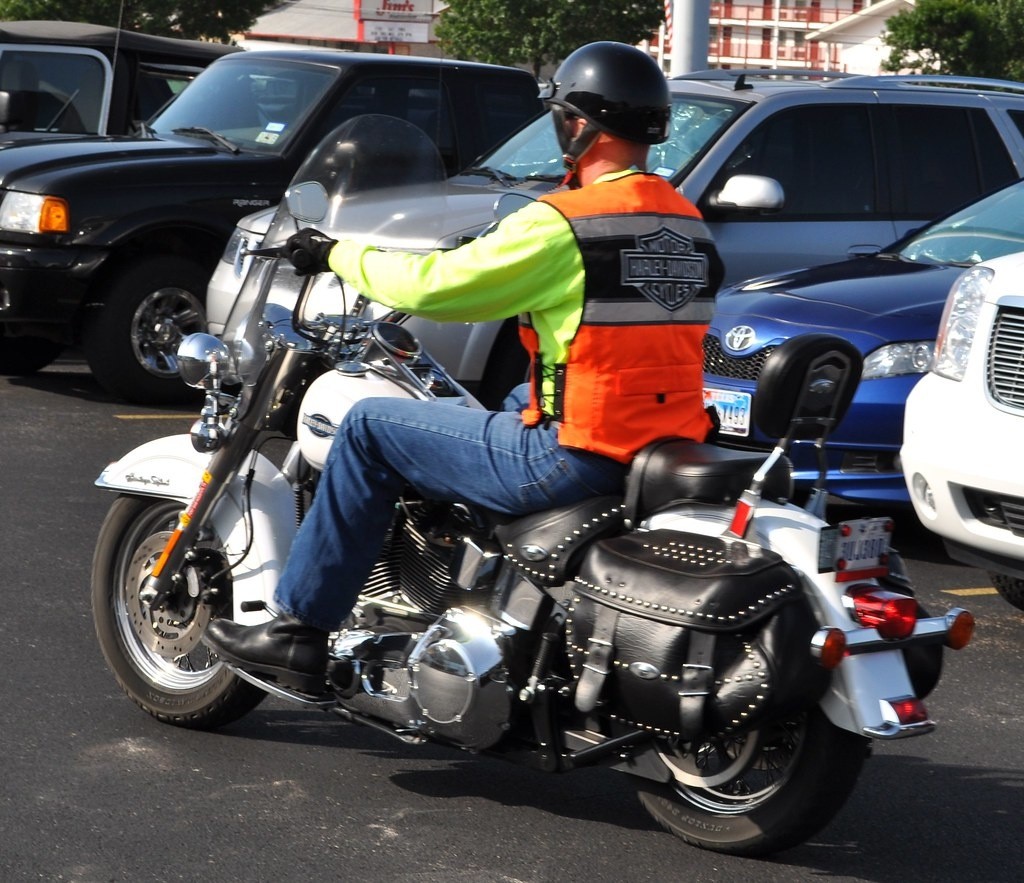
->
[201,609,329,695]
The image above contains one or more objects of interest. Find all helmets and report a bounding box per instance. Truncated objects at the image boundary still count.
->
[538,41,673,145]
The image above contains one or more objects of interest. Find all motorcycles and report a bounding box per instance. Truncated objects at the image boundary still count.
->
[92,108,974,853]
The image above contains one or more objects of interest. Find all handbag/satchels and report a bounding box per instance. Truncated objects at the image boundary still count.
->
[563,528,821,743]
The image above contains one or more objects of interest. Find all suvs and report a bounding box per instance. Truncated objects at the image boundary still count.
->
[202,68,1024,412]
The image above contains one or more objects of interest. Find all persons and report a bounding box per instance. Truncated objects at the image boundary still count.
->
[198,40,724,696]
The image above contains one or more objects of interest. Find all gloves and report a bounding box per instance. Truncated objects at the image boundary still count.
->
[285,228,338,272]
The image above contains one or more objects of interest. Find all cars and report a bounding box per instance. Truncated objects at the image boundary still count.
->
[1,15,248,136]
[3,49,539,393]
[698,181,1022,518]
[907,251,1024,607]
